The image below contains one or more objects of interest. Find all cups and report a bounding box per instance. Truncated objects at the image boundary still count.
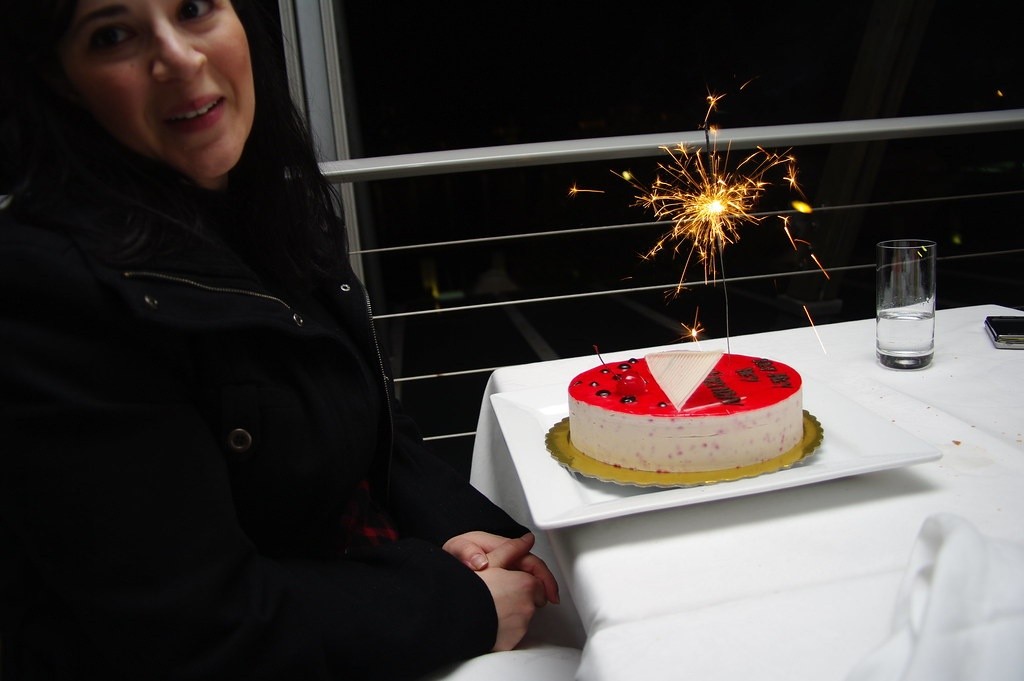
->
[876,239,936,372]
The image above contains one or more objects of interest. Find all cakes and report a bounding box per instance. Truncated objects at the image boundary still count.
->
[568,352,803,473]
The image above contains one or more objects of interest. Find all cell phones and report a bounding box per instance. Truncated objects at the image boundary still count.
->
[984,315,1024,349]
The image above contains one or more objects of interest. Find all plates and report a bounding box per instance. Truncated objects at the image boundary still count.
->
[490,347,943,532]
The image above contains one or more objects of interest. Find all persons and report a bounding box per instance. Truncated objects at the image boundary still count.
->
[0,0,560,681]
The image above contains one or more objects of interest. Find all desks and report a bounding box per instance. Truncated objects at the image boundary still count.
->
[470,305,1023,681]
